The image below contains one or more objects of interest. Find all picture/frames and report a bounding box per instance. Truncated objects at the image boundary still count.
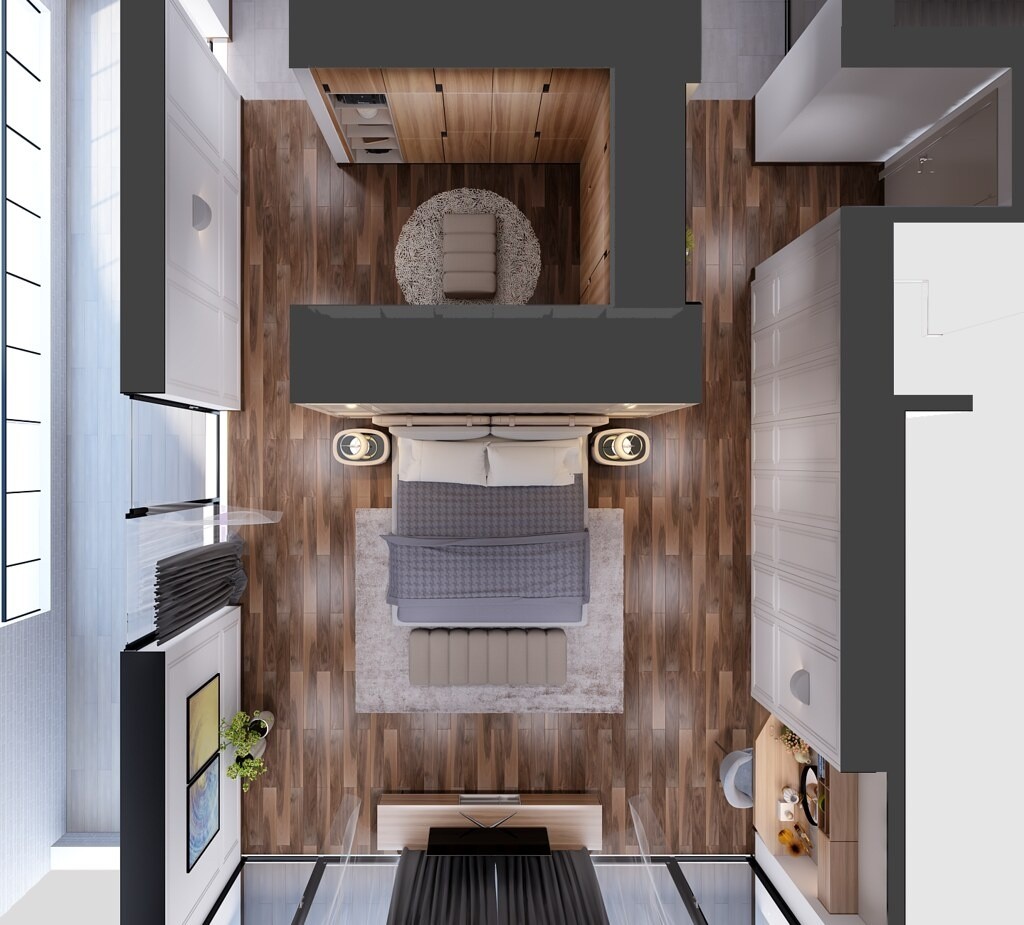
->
[186,753,220,873]
[185,672,220,784]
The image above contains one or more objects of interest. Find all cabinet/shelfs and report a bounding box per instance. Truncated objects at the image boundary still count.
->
[816,829,859,914]
[579,68,611,305]
[817,754,858,841]
[376,793,604,851]
[750,205,894,774]
[315,68,609,164]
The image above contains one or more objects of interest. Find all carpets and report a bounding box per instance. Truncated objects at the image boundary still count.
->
[353,507,626,714]
[393,186,542,306]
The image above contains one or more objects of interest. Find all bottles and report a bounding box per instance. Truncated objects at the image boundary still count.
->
[781,785,799,804]
[795,749,811,764]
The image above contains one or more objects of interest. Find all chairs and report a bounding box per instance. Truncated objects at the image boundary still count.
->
[714,741,755,809]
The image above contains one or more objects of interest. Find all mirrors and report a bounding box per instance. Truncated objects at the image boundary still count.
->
[801,765,819,827]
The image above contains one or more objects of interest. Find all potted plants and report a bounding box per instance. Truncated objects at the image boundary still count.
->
[226,738,267,792]
[218,708,275,759]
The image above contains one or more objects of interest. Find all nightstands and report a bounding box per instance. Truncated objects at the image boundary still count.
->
[333,428,391,467]
[589,427,651,467]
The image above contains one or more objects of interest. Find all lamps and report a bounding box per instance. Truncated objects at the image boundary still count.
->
[603,433,643,460]
[341,433,378,460]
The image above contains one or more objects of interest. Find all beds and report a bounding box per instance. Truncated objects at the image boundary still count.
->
[372,415,609,627]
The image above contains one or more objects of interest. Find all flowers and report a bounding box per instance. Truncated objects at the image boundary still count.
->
[770,725,810,754]
[786,838,805,858]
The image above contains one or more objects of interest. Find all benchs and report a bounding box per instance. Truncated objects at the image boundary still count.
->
[408,628,567,687]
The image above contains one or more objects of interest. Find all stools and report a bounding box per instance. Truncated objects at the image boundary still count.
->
[442,213,498,300]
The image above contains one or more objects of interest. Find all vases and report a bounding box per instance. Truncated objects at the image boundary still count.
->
[781,786,799,804]
[794,749,811,765]
[778,830,794,845]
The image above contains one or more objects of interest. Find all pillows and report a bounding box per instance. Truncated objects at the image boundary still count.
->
[399,438,489,488]
[486,439,580,486]
